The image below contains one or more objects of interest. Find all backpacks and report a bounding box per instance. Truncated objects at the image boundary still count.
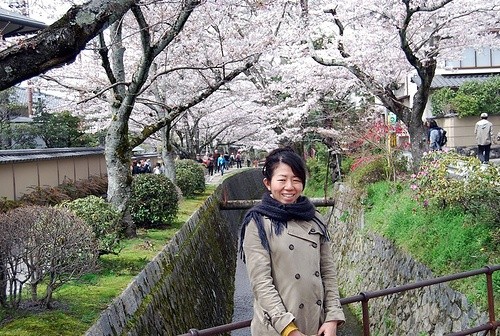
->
[429,127,447,147]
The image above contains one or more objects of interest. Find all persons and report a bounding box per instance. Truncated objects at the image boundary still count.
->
[213,150,220,173]
[153,163,164,176]
[246,152,252,167]
[229,153,236,168]
[426,118,447,152]
[217,154,226,176]
[206,153,214,177]
[131,156,152,175]
[473,112,495,165]
[238,146,345,336]
[223,152,230,170]
[235,151,242,168]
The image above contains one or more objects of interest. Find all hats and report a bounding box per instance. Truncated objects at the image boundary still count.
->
[480,112,488,118]
